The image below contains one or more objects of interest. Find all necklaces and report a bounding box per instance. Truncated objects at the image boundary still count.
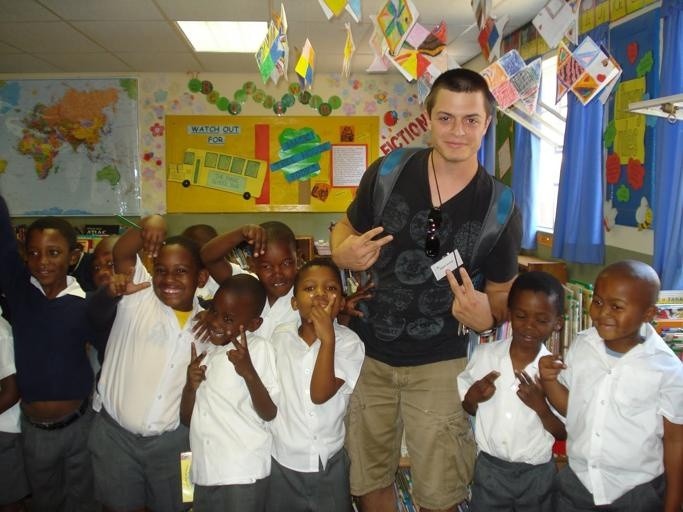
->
[430,143,481,282]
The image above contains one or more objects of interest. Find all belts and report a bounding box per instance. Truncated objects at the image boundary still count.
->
[28,399,89,431]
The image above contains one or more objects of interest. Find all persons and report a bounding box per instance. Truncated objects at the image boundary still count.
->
[455,270,566,512]
[263,255,365,511]
[86,215,214,512]
[92,232,121,290]
[537,258,681,512]
[1,194,86,512]
[199,221,373,511]
[172,223,220,345]
[1,316,28,512]
[329,67,524,512]
[14,222,30,260]
[180,272,279,511]
[67,240,92,293]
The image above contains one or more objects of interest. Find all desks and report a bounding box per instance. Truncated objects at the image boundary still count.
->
[288,235,569,290]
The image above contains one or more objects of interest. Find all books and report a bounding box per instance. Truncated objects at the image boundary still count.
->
[466,280,681,359]
[71,234,118,254]
[312,237,362,295]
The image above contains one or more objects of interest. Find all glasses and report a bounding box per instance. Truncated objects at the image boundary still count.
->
[424,207,441,259]
